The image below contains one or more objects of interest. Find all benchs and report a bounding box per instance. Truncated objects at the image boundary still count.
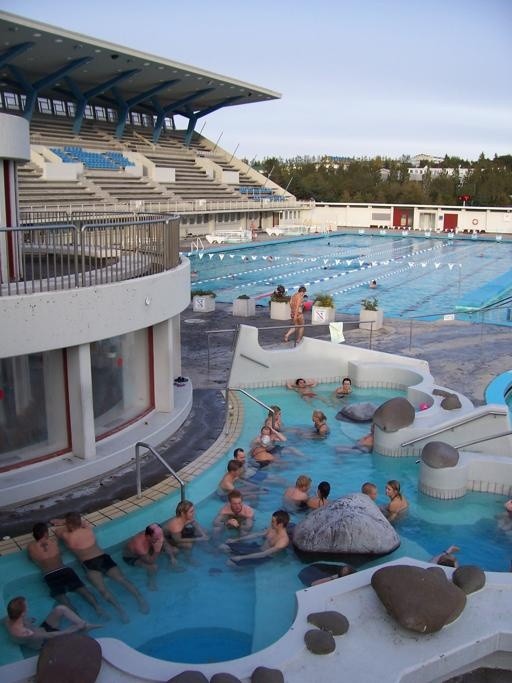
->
[1,106,296,220]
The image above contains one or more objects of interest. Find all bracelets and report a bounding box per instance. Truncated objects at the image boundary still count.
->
[443,551,448,555]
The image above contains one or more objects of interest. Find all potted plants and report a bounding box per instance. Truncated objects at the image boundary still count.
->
[190,289,383,330]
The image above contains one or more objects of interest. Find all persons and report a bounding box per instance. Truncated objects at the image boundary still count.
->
[24,520,111,625]
[430,546,459,567]
[311,567,355,585]
[242,256,249,263]
[287,379,314,388]
[286,475,330,508]
[0,595,104,651]
[362,479,407,520]
[266,256,273,263]
[337,378,352,393]
[121,520,185,592]
[505,500,512,511]
[48,508,150,623]
[163,498,212,566]
[283,286,306,345]
[367,279,377,288]
[360,434,373,445]
[216,406,289,562]
[190,268,199,277]
[313,411,328,433]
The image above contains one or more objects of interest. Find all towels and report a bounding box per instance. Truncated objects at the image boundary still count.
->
[328,320,346,344]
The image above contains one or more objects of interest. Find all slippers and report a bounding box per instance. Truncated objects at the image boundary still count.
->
[174,377,188,382]
